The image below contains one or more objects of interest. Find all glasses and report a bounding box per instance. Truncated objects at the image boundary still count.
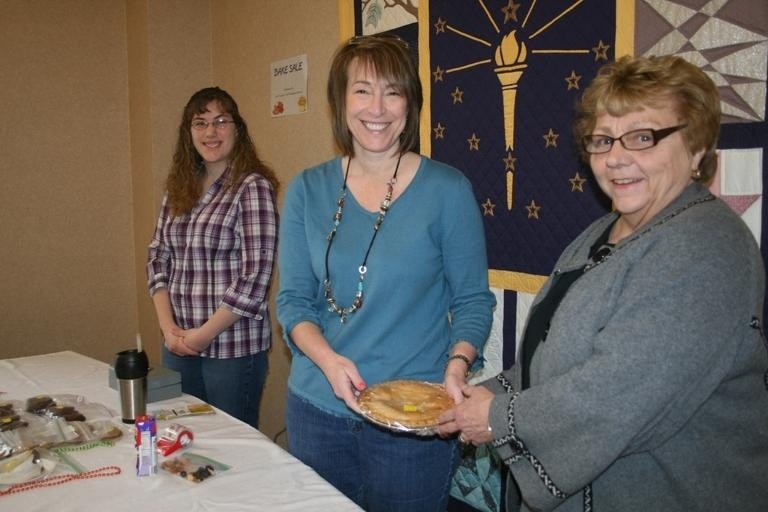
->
[190,118,234,132]
[577,121,687,158]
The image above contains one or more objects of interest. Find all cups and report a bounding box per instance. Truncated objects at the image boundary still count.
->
[115,350,149,423]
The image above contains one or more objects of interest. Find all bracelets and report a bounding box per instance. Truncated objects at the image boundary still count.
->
[443,354,471,379]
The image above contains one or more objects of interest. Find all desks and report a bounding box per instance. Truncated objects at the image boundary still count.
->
[0,349,367,512]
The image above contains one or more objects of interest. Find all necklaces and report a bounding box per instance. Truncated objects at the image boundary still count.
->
[53,439,112,455]
[582,193,717,272]
[322,149,402,322]
[0,466,120,497]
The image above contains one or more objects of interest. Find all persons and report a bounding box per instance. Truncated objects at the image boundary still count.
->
[145,85,282,429]
[273,32,498,511]
[431,51,768,512]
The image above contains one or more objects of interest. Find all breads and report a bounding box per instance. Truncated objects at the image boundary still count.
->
[358,380,457,427]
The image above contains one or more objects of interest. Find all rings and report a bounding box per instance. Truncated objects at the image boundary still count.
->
[458,432,467,443]
